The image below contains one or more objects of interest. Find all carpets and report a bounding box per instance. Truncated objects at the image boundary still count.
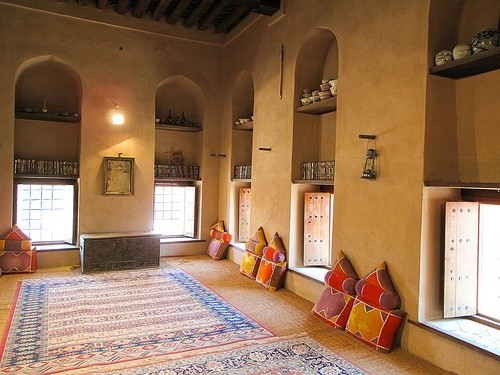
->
[0,263,278,375]
[47,331,373,375]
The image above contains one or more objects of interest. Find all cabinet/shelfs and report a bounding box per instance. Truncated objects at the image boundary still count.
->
[232,121,253,182]
[424,48,500,190]
[154,123,203,181]
[13,112,81,179]
[294,96,336,185]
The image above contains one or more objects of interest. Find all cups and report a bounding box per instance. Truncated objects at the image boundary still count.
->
[156,119,160,123]
[300,98,312,106]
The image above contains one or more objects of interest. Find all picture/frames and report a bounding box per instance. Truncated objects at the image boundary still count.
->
[103,157,135,195]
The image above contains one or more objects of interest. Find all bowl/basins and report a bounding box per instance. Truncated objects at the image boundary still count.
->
[318,78,338,101]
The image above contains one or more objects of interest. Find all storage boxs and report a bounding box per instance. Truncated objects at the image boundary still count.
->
[79,232,162,274]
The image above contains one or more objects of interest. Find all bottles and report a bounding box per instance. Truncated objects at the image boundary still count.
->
[181,111,187,126]
[166,109,172,125]
[176,114,179,125]
